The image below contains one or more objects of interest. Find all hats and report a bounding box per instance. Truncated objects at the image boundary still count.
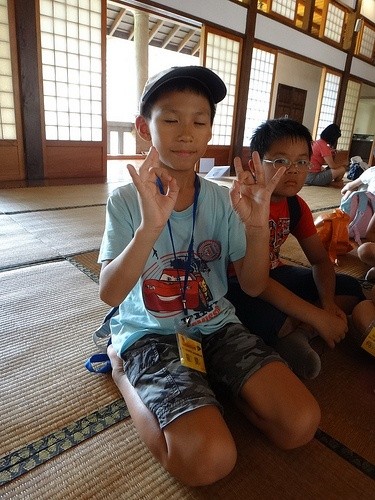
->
[139,65,228,115]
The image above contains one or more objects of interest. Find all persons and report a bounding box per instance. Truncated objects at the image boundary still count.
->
[97,65,321,486]
[224,116,364,380]
[306,123,346,187]
[339,138,375,336]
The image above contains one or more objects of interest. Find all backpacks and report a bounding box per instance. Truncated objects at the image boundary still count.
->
[339,191,375,247]
[313,210,354,265]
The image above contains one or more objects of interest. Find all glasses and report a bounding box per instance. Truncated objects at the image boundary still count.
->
[262,157,313,172]
